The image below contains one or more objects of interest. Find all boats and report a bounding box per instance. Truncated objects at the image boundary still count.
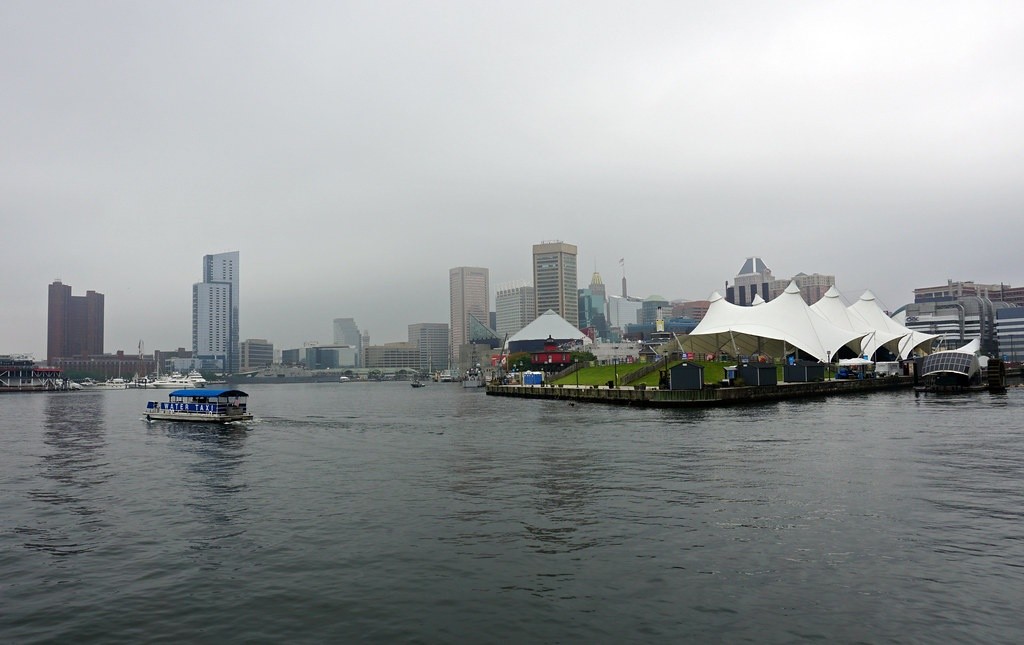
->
[184,369,207,387]
[142,388,253,422]
[153,379,194,388]
[70,377,160,390]
[410,379,426,387]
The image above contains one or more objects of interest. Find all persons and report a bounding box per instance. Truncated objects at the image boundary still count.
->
[232,397,239,408]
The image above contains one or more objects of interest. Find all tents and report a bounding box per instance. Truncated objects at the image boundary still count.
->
[838,358,874,374]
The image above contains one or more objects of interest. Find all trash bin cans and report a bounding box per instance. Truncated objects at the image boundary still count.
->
[540,380,544,387]
[608,381,613,388]
